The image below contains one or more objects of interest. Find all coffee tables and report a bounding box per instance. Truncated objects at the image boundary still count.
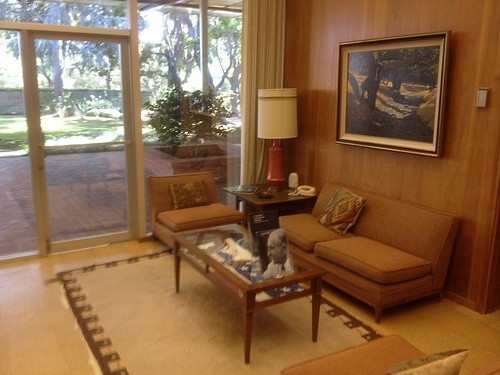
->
[173,222,327,365]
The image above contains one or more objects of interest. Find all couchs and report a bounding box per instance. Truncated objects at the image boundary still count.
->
[277,182,459,323]
[147,170,244,250]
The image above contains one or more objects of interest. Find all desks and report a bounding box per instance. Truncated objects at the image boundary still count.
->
[222,179,317,239]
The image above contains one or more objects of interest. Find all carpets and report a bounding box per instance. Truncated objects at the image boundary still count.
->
[58,231,385,375]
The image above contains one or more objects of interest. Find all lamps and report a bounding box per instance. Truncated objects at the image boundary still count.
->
[255,87,300,186]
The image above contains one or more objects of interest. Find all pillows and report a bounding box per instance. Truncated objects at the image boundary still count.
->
[385,347,470,375]
[317,185,368,238]
[167,180,210,212]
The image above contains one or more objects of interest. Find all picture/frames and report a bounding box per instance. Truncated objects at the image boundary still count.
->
[333,28,453,160]
